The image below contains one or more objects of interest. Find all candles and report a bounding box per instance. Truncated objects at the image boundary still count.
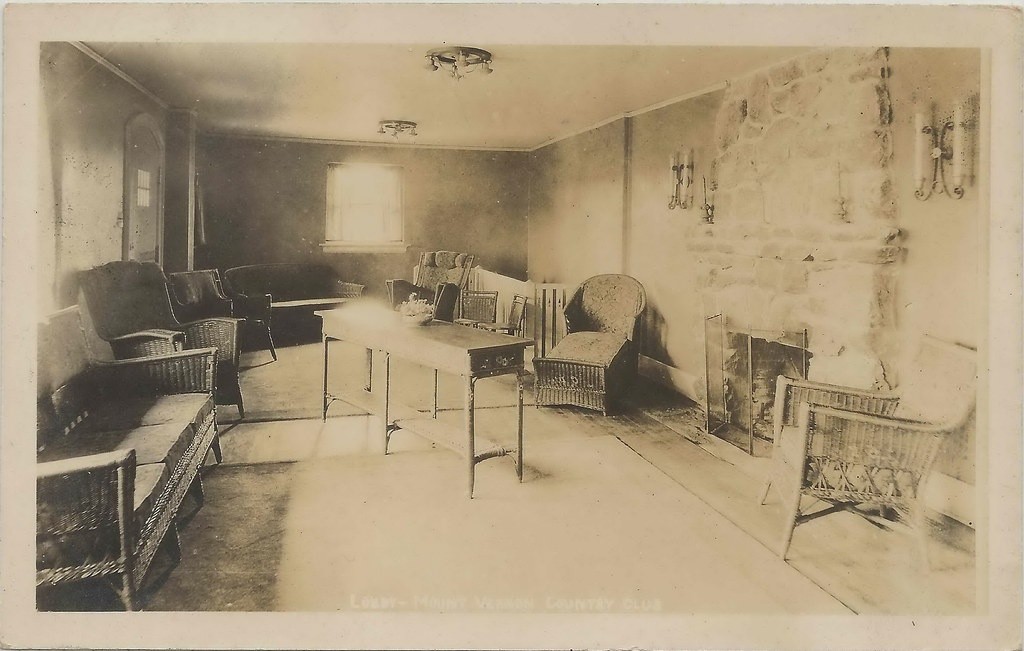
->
[914,114,924,181]
[954,109,964,185]
[670,155,675,197]
[686,153,692,197]
[702,174,707,204]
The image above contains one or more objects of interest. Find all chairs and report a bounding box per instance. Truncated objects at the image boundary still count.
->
[453,289,498,332]
[76,258,245,421]
[478,294,528,336]
[169,268,278,363]
[385,251,475,321]
[531,274,647,417]
[759,333,978,572]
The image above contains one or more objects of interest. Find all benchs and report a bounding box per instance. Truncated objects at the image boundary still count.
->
[223,262,367,344]
[37,304,222,612]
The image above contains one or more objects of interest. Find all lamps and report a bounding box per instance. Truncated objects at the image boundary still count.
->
[424,46,494,81]
[377,120,418,140]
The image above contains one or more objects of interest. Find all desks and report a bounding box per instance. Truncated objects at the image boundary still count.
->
[314,307,535,499]
